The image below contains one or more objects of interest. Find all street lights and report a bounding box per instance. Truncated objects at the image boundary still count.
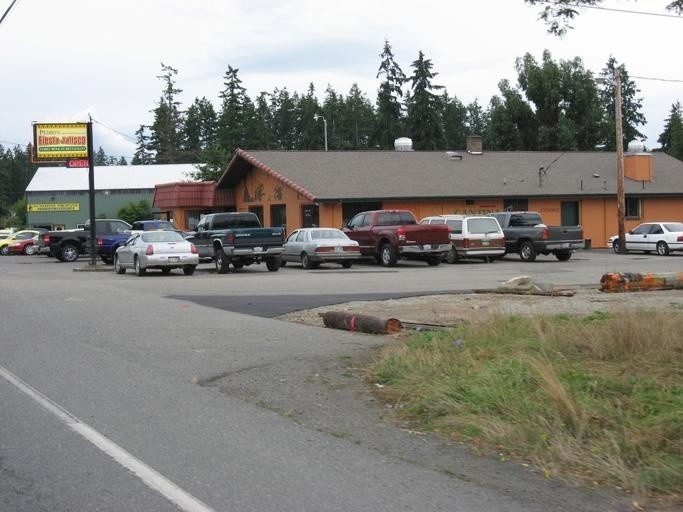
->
[594,76,626,253]
[312,114,327,151]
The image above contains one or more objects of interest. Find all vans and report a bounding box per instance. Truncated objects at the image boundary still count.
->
[417,215,505,264]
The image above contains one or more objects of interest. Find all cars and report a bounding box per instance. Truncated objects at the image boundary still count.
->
[605,222,683,256]
[280,227,361,269]
[0,218,198,277]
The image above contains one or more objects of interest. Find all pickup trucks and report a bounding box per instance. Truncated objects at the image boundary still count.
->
[184,212,286,275]
[338,210,453,266]
[485,211,585,262]
